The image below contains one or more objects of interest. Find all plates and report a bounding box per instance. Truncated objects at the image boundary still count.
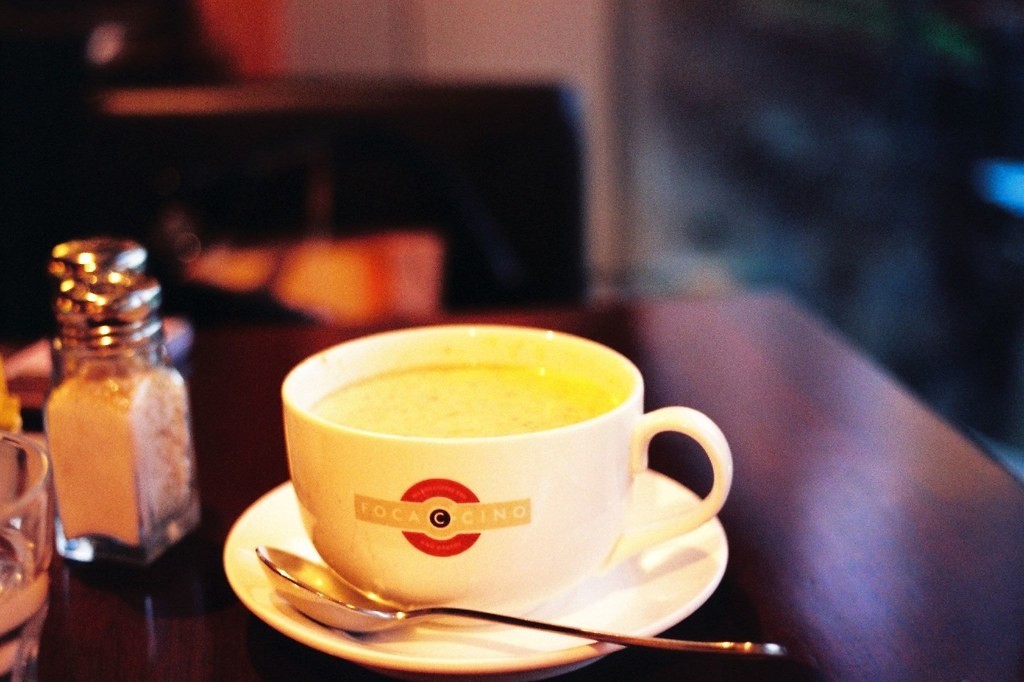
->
[224,470,730,682]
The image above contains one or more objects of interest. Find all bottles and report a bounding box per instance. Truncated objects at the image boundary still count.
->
[43,239,200,562]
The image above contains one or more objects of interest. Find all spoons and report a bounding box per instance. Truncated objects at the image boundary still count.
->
[255,546,786,656]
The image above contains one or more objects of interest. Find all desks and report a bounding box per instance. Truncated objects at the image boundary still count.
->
[0,287,1022,682]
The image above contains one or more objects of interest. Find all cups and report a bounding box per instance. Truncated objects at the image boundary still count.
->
[281,323,733,628]
[0,432,50,682]
